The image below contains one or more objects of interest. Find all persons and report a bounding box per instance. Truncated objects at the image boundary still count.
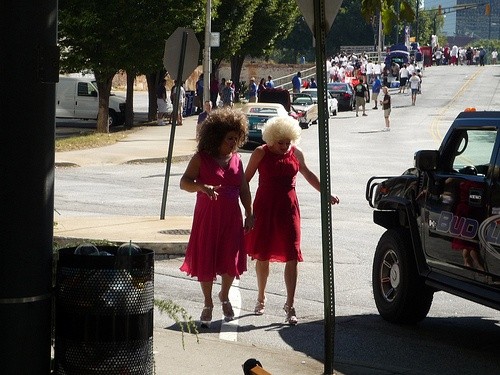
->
[195,100,213,140]
[379,88,391,128]
[292,73,302,94]
[181,108,253,327]
[266,75,274,89]
[492,50,498,64]
[352,78,368,116]
[304,47,422,108]
[157,79,168,125]
[171,80,185,125]
[431,43,486,66]
[258,78,267,97]
[245,123,339,325]
[196,74,236,107]
[300,56,305,65]
[238,77,257,103]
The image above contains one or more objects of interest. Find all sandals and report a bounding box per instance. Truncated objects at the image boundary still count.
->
[219,295,234,322]
[199,305,213,327]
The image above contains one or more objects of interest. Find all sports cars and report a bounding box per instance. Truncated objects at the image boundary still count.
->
[288,92,319,129]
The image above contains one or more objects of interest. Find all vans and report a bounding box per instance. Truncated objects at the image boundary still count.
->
[385,50,411,69]
[56,74,130,130]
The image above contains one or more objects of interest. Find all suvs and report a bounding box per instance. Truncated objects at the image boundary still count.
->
[364,107,500,328]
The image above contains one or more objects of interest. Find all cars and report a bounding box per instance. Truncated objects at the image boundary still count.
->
[239,102,289,145]
[300,89,339,118]
[327,82,358,111]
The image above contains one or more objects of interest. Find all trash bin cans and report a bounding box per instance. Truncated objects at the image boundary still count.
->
[55,243,157,375]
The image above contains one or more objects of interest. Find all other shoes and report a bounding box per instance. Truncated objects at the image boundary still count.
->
[284,305,299,324]
[254,297,266,315]
